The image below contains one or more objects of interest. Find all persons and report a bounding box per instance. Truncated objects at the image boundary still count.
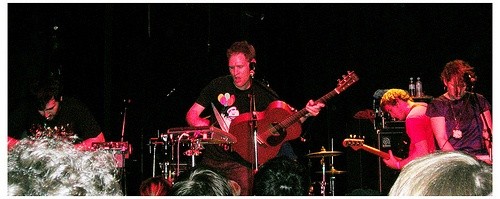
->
[187,41,325,161]
[424,59,492,164]
[230,179,241,195]
[140,176,170,196]
[169,164,237,196]
[21,77,105,151]
[253,157,313,196]
[8,122,124,196]
[388,150,493,196]
[380,88,441,169]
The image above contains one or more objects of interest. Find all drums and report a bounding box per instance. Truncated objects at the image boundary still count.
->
[159,162,191,184]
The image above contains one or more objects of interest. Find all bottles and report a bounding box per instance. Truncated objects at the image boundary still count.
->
[409,78,415,96]
[416,78,422,97]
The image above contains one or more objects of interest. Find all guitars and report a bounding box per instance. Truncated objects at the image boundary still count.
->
[343,135,404,162]
[228,70,359,167]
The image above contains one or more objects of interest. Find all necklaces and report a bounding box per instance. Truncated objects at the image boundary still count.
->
[447,93,471,138]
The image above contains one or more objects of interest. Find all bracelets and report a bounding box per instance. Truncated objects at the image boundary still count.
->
[397,160,402,169]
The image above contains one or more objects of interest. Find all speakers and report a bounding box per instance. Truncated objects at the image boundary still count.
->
[377,130,411,193]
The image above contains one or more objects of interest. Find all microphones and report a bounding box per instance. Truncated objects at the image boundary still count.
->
[249,57,256,80]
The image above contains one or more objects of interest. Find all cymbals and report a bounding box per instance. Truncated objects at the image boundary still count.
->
[306,151,341,158]
[320,169,346,173]
